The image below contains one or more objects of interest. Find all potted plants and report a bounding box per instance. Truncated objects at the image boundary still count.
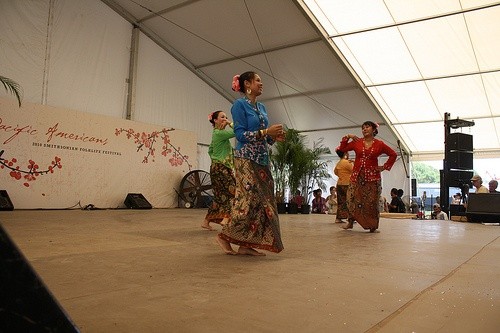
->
[285,143,314,213]
[299,146,332,214]
[269,129,306,214]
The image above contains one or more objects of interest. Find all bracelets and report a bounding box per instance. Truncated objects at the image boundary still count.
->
[258,130,264,138]
[230,122,234,127]
[265,129,268,135]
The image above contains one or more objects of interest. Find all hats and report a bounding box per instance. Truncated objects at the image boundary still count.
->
[470,176,481,180]
[454,193,461,198]
[434,203,440,208]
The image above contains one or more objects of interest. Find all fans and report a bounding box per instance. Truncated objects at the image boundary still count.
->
[180,170,213,208]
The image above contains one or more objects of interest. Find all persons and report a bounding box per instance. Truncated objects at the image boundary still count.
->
[488,180,500,193]
[333,146,358,223]
[432,196,449,220]
[470,175,490,194]
[289,190,305,207]
[201,110,236,232]
[339,120,397,233]
[311,186,406,215]
[452,192,463,204]
[215,71,287,256]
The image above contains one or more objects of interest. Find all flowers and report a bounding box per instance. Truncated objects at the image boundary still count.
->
[232,74,241,90]
[208,112,215,119]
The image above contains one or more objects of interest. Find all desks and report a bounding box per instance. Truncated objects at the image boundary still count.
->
[380,213,417,220]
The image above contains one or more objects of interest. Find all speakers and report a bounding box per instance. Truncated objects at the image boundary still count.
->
[447,132,474,189]
[0,190,14,211]
[124,193,152,209]
[465,192,500,224]
[277,202,298,215]
